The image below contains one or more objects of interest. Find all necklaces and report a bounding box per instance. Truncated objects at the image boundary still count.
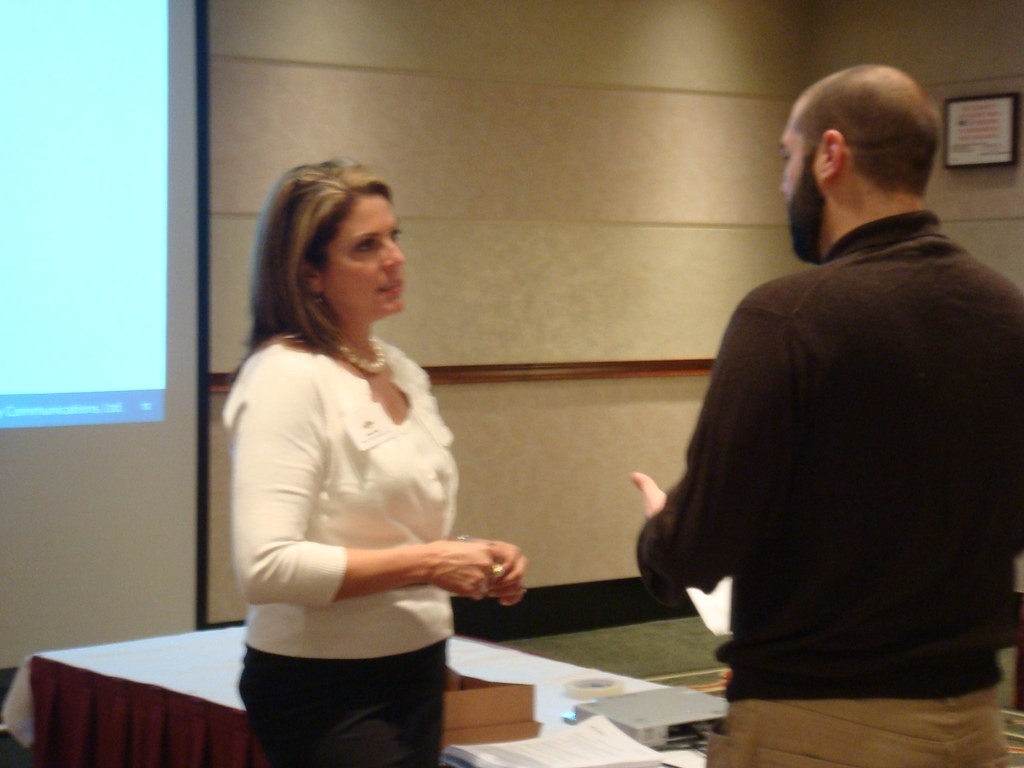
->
[337,337,384,374]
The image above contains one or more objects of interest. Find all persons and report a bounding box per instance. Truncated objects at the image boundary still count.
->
[220,154,523,768]
[630,66,1024,768]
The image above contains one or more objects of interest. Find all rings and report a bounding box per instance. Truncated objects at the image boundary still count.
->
[492,565,505,576]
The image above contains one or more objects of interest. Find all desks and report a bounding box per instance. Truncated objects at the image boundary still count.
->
[0,624,706,768]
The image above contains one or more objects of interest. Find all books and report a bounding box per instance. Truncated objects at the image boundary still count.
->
[445,715,662,768]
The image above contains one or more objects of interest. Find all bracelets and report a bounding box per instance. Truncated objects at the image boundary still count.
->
[456,534,469,540]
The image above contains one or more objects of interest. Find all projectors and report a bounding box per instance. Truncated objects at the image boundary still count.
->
[573,683,731,749]
